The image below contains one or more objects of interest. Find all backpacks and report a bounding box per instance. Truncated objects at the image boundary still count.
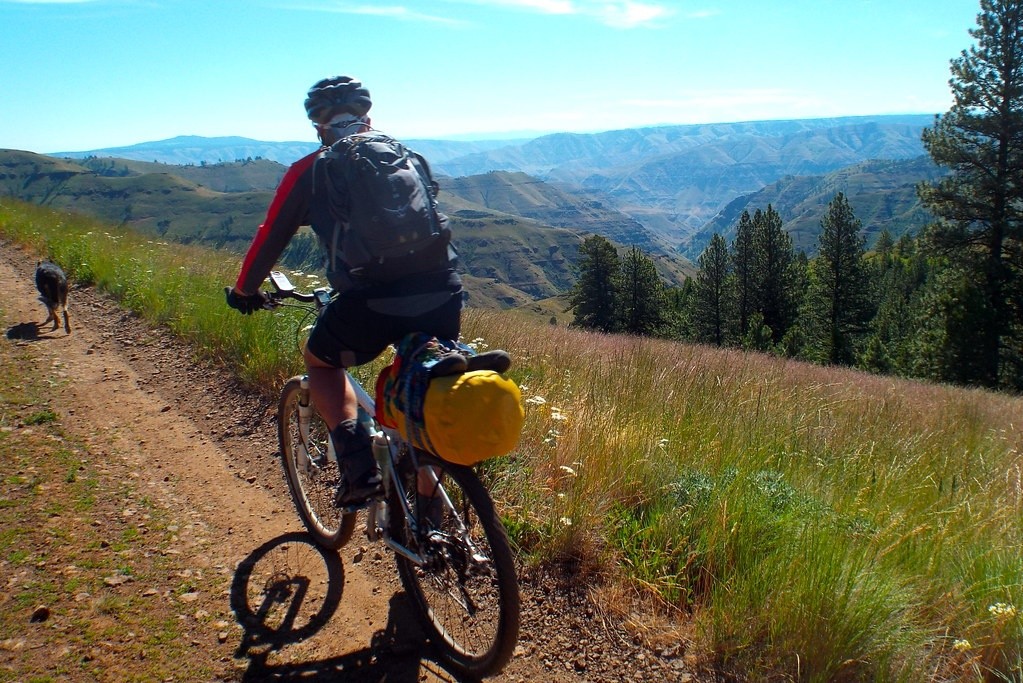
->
[309,122,453,294]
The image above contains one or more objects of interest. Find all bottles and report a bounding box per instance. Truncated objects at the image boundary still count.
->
[358,405,376,438]
[371,431,391,480]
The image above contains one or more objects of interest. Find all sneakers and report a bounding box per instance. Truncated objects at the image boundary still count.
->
[435,331,511,374]
[399,331,469,376]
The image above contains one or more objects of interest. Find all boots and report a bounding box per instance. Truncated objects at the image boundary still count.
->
[335,416,385,505]
[414,492,447,574]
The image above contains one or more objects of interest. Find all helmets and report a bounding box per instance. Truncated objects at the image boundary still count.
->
[304,75,373,124]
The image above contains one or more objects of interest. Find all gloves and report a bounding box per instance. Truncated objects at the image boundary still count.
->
[230,287,266,316]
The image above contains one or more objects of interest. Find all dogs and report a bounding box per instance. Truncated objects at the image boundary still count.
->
[35,259,71,334]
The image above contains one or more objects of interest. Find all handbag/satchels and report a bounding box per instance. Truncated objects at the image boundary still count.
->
[374,343,523,465]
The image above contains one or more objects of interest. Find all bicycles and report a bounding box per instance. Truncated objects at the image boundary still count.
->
[224,270,521,678]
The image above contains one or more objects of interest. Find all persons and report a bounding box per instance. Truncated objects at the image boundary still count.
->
[224,75,465,530]
